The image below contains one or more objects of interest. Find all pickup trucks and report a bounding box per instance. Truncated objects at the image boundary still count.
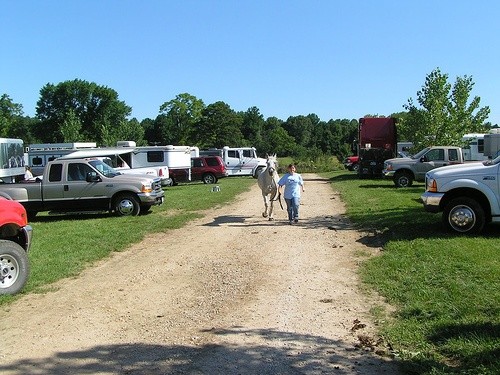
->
[382,132,500,236]
[0,195,33,298]
[0,157,165,217]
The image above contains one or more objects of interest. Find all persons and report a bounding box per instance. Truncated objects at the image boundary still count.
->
[278,164,304,224]
[25,167,32,180]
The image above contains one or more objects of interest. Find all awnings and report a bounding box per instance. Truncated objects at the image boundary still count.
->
[66,149,133,167]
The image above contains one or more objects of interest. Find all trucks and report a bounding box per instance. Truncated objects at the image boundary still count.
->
[0,137,269,187]
[357,117,398,179]
[344,156,359,171]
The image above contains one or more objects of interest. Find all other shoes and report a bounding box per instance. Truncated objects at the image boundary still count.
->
[294,217,298,223]
[288,220,293,225]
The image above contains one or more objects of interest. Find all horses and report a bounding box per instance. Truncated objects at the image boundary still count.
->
[257,153,283,222]
